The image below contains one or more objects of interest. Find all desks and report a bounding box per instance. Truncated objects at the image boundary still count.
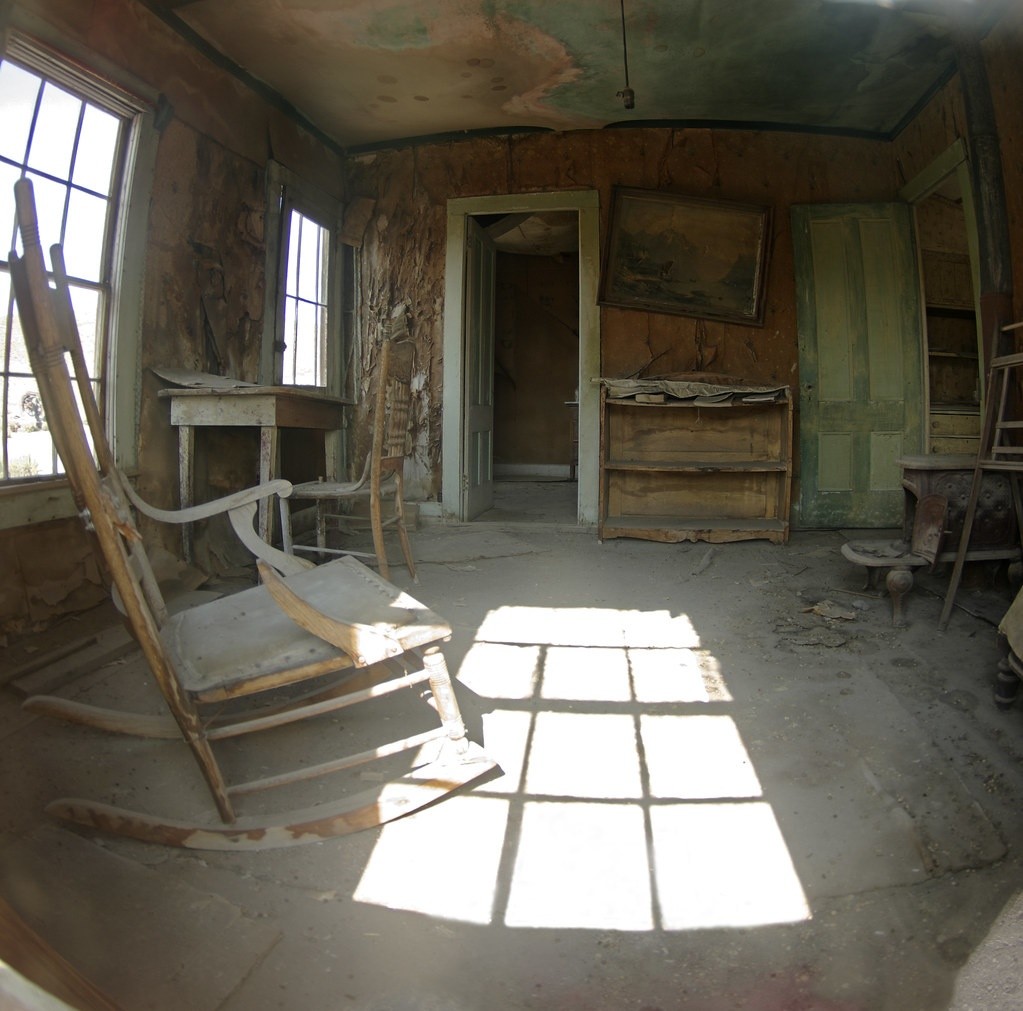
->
[157,386,355,588]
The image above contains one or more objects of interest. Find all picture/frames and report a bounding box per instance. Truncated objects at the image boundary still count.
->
[594,184,776,329]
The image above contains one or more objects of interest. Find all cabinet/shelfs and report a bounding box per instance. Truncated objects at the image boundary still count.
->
[597,370,793,545]
[920,248,981,455]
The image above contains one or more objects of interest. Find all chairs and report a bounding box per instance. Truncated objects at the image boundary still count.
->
[9,180,499,850]
[280,336,417,582]
